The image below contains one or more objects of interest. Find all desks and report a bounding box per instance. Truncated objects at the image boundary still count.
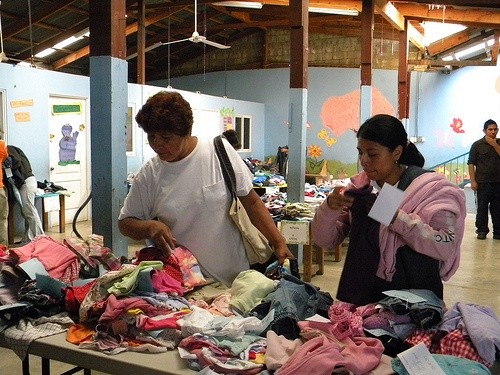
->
[35,191,75,233]
[0,313,198,375]
[253,174,343,282]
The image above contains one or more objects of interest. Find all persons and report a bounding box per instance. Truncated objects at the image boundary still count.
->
[0,139,45,248]
[118,91,295,288]
[467,119,500,240]
[309,115,457,307]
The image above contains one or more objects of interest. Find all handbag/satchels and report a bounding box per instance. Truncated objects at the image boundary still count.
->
[229,194,276,265]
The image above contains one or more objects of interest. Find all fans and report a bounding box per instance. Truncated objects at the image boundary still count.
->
[0,0,48,71]
[154,0,231,50]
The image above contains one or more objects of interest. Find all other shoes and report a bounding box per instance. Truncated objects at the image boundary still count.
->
[493,235,500,239]
[477,232,487,239]
